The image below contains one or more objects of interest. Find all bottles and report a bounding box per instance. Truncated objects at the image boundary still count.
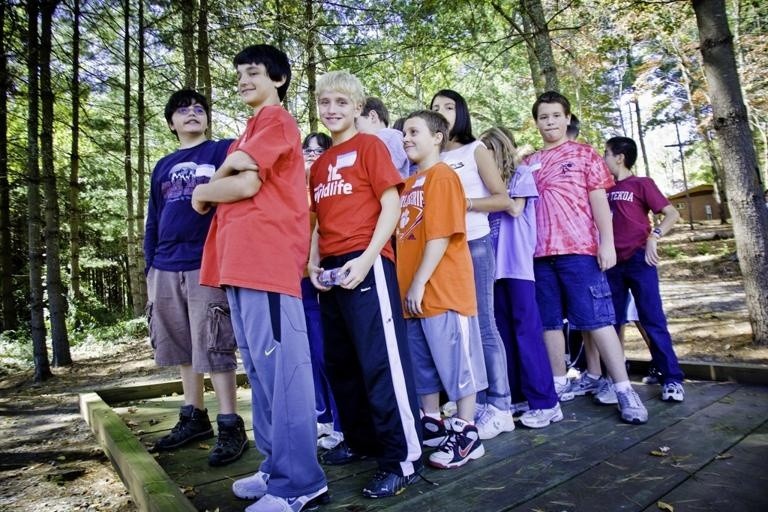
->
[318,266,351,288]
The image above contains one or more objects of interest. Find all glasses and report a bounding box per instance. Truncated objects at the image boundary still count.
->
[174,106,208,115]
[302,147,326,155]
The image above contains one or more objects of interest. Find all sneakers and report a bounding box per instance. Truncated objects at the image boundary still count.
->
[361,462,426,500]
[419,365,632,470]
[154,405,216,455]
[232,470,331,511]
[208,413,250,469]
[311,421,368,466]
[613,385,650,424]
[662,381,686,402]
[641,372,665,386]
